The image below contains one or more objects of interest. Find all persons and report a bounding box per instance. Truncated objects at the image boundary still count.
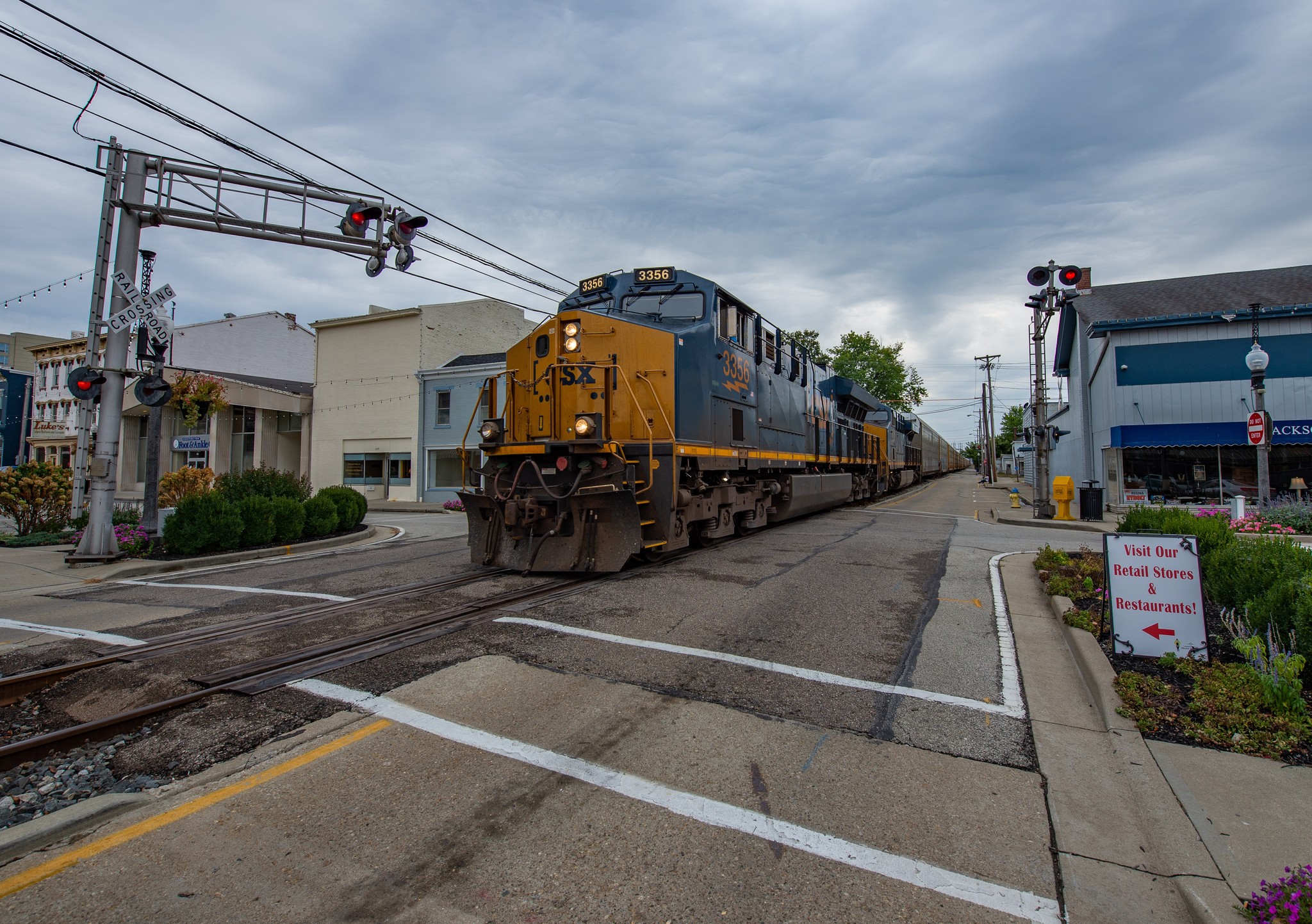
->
[977,474,989,483]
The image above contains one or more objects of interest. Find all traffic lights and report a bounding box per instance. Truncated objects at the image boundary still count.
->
[1025,302,1041,316]
[344,204,383,233]
[68,367,107,399]
[978,446,981,448]
[134,376,168,405]
[1027,266,1050,287]
[1035,425,1046,443]
[396,213,428,239]
[1058,296,1074,309]
[1058,265,1082,286]
[1015,427,1031,444]
[1054,427,1071,443]
[973,446,975,448]
[1062,291,1080,302]
[1029,288,1047,309]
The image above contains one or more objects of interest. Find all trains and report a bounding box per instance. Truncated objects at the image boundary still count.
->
[455,260,971,576]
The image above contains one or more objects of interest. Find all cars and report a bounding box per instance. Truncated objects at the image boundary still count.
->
[1191,479,1277,502]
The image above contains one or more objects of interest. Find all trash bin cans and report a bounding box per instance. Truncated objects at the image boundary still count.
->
[1075,479,1106,522]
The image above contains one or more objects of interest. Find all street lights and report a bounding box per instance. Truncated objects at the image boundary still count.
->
[982,449,985,459]
[984,438,987,459]
[967,414,987,476]
[1245,339,1270,511]
[969,433,980,444]
[90,421,98,457]
[1017,451,1021,476]
[977,452,980,472]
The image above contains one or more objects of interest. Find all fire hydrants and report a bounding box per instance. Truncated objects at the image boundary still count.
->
[1008,487,1021,508]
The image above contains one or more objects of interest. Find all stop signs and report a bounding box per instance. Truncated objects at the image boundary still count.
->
[1247,411,1265,445]
[983,459,987,464]
